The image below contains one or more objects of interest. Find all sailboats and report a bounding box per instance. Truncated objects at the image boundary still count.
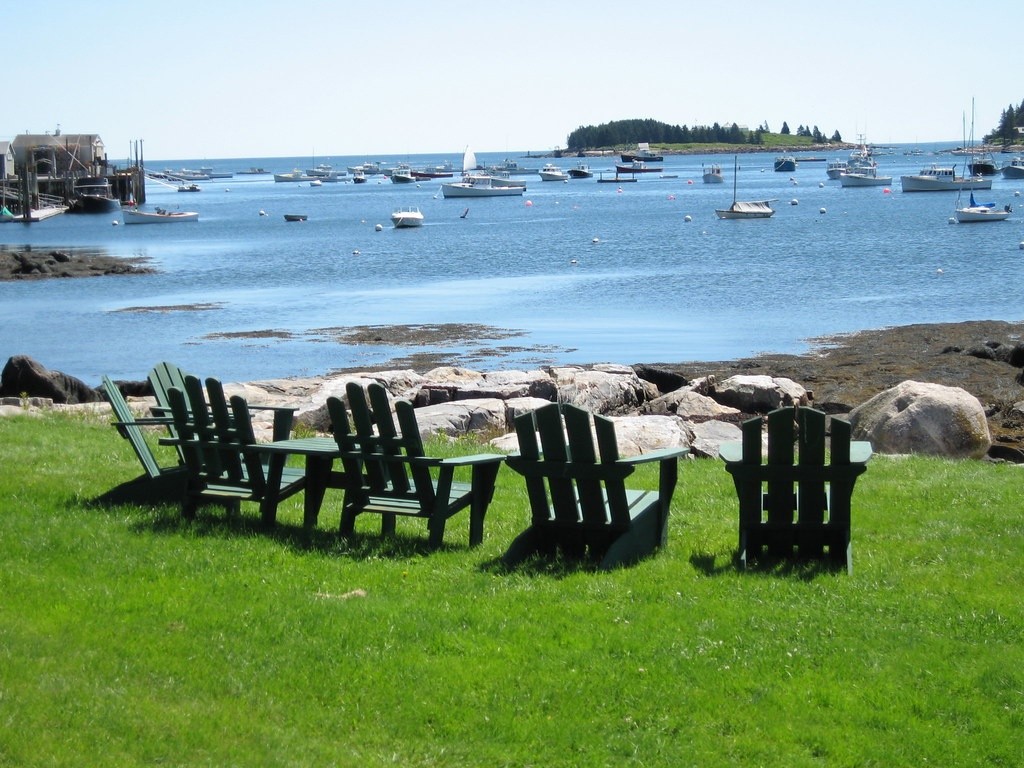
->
[954,98,1009,223]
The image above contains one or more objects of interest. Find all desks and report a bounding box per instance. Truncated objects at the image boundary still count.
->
[245,438,360,527]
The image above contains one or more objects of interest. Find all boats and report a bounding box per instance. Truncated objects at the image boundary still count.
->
[620,145,663,162]
[441,173,528,198]
[178,187,202,192]
[284,214,308,221]
[901,165,992,192]
[965,138,1004,177]
[999,156,1024,179]
[122,209,199,225]
[773,150,799,172]
[714,154,779,219]
[389,206,425,227]
[566,159,595,179]
[162,144,539,186]
[538,164,568,181]
[615,158,664,173]
[824,133,893,189]
[701,161,724,183]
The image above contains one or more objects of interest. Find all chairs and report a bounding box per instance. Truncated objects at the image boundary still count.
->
[716,403,873,573]
[99,359,506,552]
[502,399,692,571]
